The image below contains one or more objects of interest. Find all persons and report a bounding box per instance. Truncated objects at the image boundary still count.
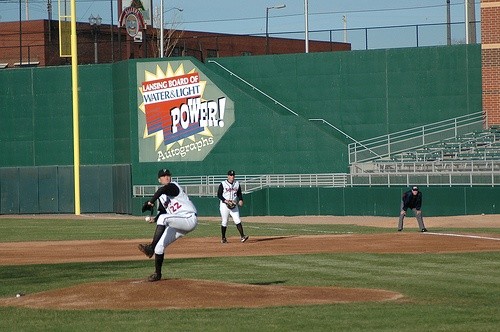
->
[138,169,197,282]
[218,170,249,243]
[398,186,428,232]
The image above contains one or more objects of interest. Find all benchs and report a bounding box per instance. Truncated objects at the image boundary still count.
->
[374,123,500,173]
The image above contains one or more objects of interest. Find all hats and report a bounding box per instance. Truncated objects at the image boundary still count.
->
[158,169,171,177]
[412,186,419,191]
[228,170,235,175]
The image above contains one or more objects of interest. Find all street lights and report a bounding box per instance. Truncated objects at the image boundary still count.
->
[88,13,102,64]
[265,4,286,55]
[155,6,184,49]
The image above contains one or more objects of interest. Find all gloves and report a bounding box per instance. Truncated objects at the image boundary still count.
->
[142,200,154,216]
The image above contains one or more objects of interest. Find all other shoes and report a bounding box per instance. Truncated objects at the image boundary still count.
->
[421,228,427,232]
[241,235,249,243]
[221,238,229,243]
[398,229,402,231]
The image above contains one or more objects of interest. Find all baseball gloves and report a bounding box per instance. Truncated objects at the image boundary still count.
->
[142,201,154,216]
[227,200,236,210]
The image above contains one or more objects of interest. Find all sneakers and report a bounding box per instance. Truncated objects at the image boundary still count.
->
[148,273,161,281]
[138,244,154,258]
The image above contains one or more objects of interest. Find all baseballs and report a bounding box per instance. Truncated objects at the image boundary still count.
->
[16,294,20,297]
[145,216,151,221]
